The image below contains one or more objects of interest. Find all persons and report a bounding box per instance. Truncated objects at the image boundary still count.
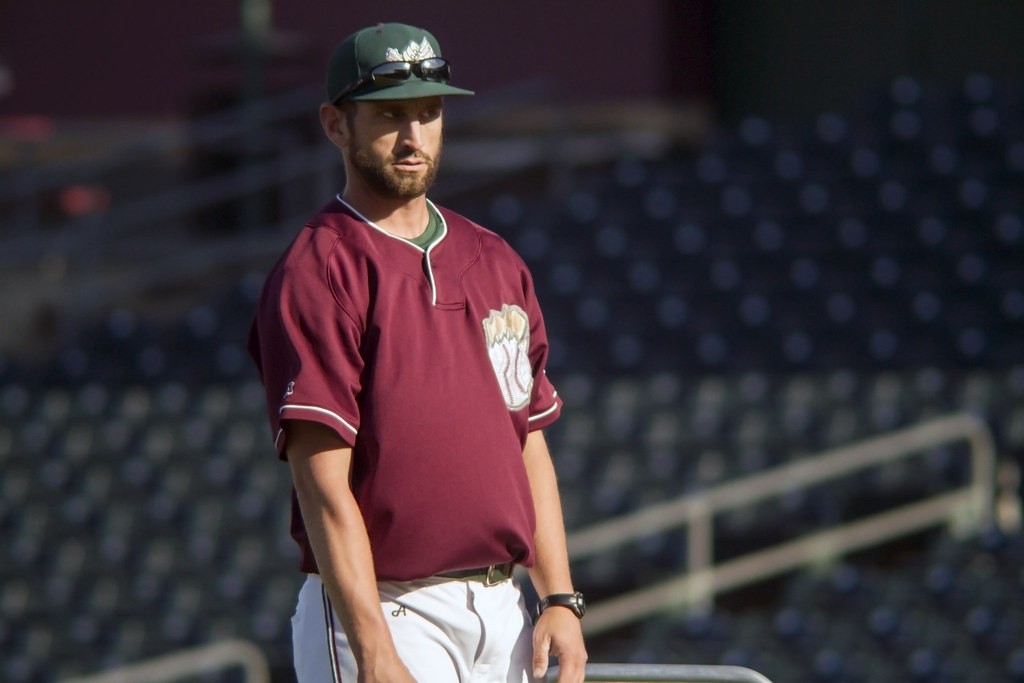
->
[246,23,588,683]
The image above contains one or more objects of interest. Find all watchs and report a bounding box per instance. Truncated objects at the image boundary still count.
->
[532,591,585,626]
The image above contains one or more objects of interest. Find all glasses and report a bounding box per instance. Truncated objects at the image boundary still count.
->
[334,58,451,98]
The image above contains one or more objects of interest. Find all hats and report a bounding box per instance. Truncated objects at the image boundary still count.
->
[329,23,476,101]
